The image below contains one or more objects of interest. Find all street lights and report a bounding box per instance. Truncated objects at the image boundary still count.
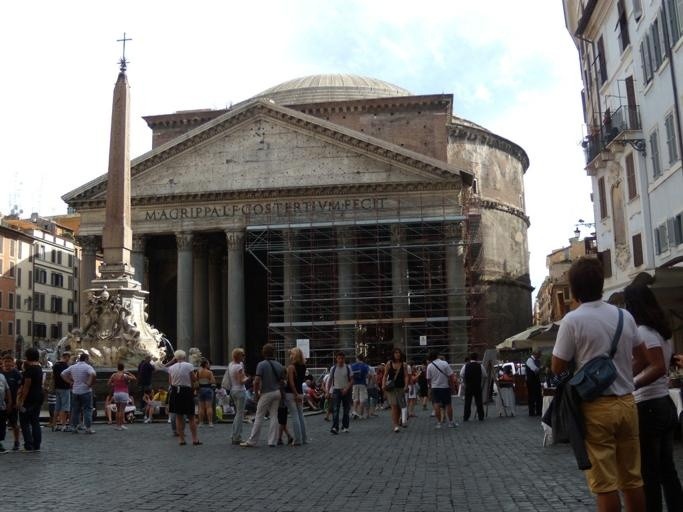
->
[566,308,624,404]
[221,369,232,390]
[385,362,403,393]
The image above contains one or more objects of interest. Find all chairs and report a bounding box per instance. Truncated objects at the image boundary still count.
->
[240,442,255,447]
[114,428,121,431]
[232,440,244,445]
[0,448,10,454]
[330,427,338,436]
[120,426,129,429]
[277,440,284,446]
[340,429,350,433]
[19,449,32,452]
[392,426,400,433]
[434,424,442,429]
[29,449,42,453]
[267,444,276,448]
[449,422,458,428]
[287,435,293,446]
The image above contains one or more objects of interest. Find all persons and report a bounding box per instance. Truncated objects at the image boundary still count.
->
[382,348,408,432]
[322,366,334,421]
[552,255,652,511]
[168,349,204,445]
[109,363,136,431]
[1,350,53,369]
[440,399,446,424]
[1,355,20,449]
[245,384,257,409]
[418,361,429,407]
[427,353,458,429]
[126,394,136,424]
[195,357,217,427]
[46,390,56,427]
[138,355,155,391]
[240,344,286,447]
[278,396,293,445]
[326,352,352,433]
[144,386,171,423]
[168,384,177,435]
[285,347,308,445]
[302,375,325,411]
[52,353,73,431]
[105,392,117,423]
[526,350,545,415]
[623,283,683,512]
[16,349,43,450]
[60,354,97,433]
[407,361,421,417]
[93,394,97,411]
[431,404,437,417]
[141,391,152,420]
[367,363,386,417]
[497,365,517,417]
[0,373,12,454]
[460,354,486,421]
[350,354,371,418]
[221,348,252,442]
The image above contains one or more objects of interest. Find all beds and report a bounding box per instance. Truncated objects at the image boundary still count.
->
[493,318,562,349]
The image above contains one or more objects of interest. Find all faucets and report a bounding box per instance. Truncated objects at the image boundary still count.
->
[192,440,203,446]
[179,442,187,446]
